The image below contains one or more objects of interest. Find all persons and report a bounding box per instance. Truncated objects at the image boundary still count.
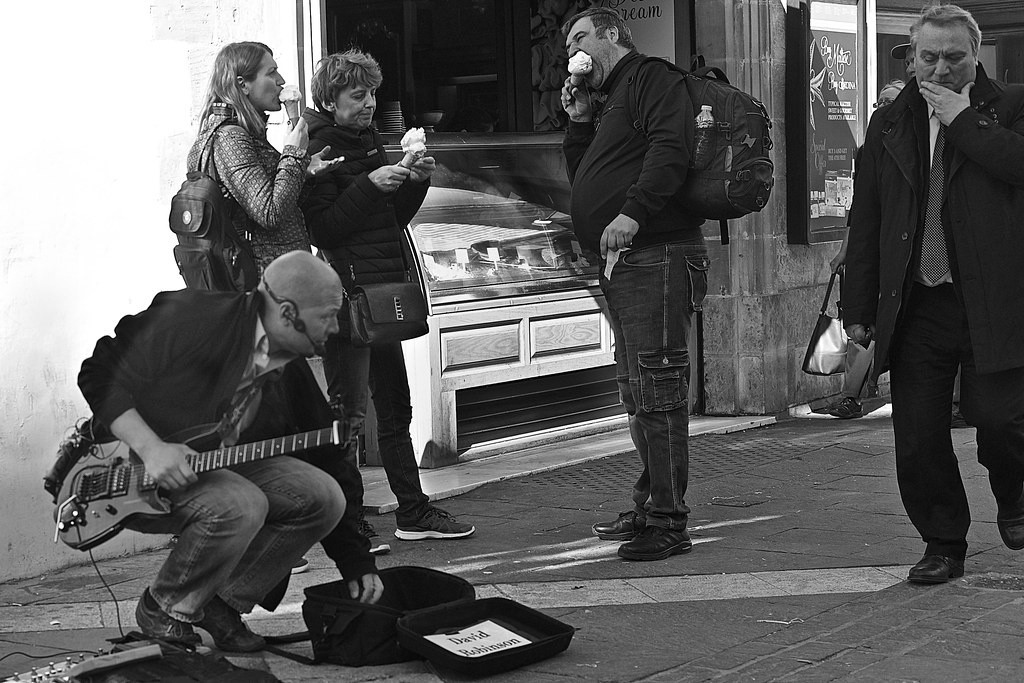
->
[301,50,476,553]
[188,42,346,572]
[843,6,1023,583]
[890,44,973,429]
[832,80,906,420]
[44,250,382,658]
[560,6,710,561]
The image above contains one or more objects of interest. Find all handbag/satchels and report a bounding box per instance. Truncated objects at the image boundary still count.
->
[345,283,430,349]
[801,264,848,377]
[300,565,574,679]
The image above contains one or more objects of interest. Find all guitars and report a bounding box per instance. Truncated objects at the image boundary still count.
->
[52,404,368,553]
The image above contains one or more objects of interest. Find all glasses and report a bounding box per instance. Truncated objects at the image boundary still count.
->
[873,99,895,108]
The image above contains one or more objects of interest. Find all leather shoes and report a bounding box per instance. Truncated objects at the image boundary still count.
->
[908,551,965,583]
[988,471,1024,551]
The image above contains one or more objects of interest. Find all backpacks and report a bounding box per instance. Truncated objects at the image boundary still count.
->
[169,118,258,293]
[625,54,774,221]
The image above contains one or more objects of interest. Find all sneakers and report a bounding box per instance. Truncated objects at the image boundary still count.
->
[949,408,973,429]
[618,525,692,560]
[290,558,307,575]
[135,588,201,646]
[358,518,390,553]
[829,397,863,419]
[395,507,476,540]
[592,511,649,541]
[193,596,265,654]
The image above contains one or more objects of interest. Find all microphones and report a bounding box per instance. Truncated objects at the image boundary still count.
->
[305,332,326,356]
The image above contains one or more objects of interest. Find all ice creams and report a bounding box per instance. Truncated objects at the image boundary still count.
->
[568,50,593,88]
[279,85,303,119]
[400,127,426,166]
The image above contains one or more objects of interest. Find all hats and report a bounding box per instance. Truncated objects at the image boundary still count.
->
[891,42,911,60]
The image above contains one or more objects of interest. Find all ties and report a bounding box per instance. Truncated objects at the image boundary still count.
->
[922,122,953,284]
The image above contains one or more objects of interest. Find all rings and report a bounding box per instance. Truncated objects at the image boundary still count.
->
[397,185,399,189]
